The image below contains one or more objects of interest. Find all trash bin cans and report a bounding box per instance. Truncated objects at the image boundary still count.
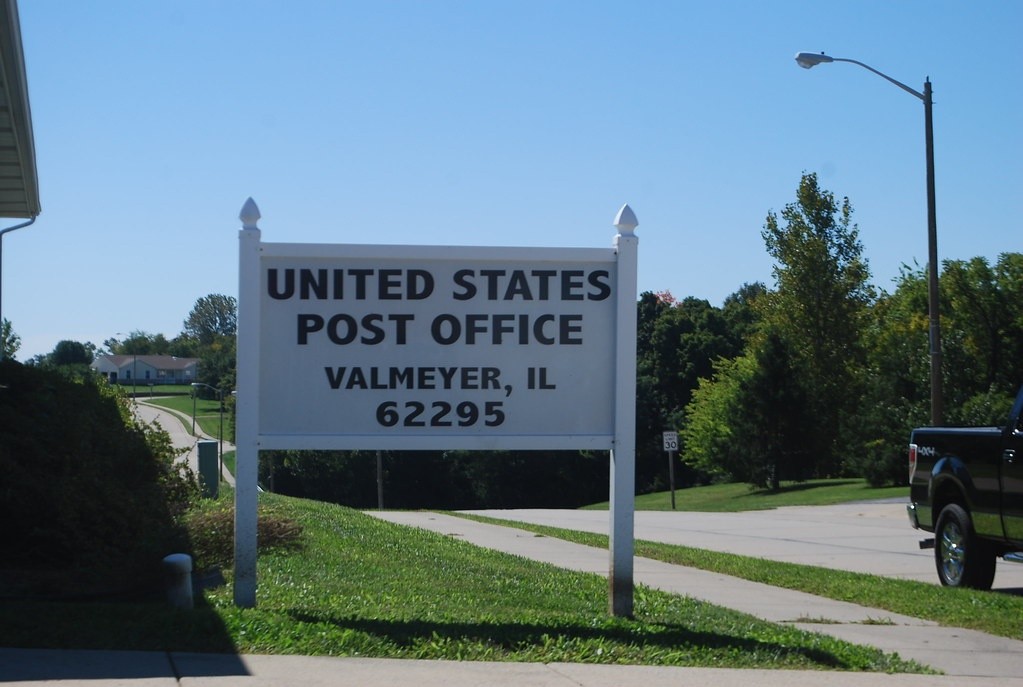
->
[197,441,219,498]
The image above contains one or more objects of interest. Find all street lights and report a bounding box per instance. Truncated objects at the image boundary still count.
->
[793,52,944,428]
[117,332,136,404]
[191,381,224,482]
[170,354,198,435]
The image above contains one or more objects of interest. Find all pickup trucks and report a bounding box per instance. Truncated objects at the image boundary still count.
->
[906,382,1023,587]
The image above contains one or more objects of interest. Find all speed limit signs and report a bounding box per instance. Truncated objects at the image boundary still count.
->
[663,430,679,453]
